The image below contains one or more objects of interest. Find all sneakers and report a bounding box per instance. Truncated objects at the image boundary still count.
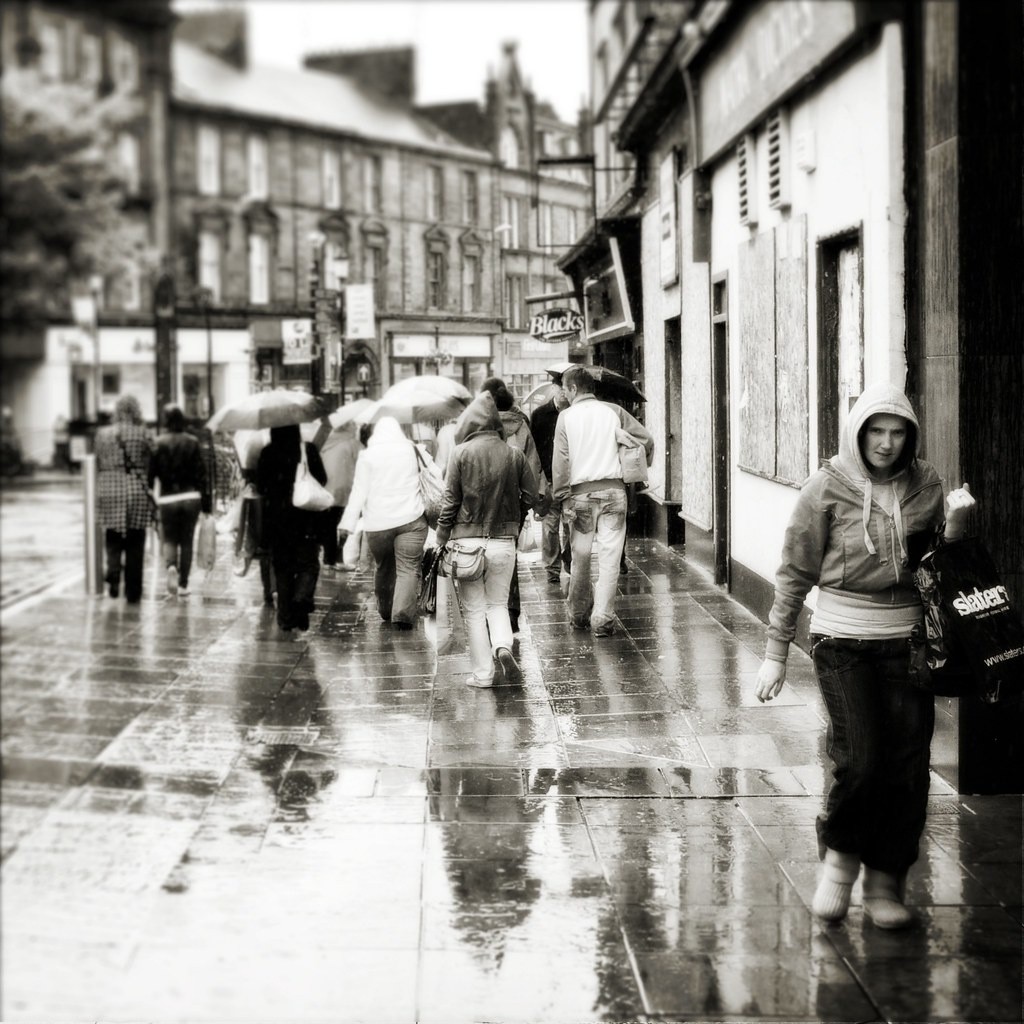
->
[466,676,494,688]
[497,648,520,680]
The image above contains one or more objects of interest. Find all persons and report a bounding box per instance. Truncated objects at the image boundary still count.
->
[92,368,655,691]
[754,384,977,928]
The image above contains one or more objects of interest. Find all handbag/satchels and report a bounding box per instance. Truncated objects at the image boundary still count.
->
[292,441,335,511]
[443,542,487,583]
[412,443,448,529]
[906,528,1018,697]
[416,547,446,614]
[425,572,466,657]
[615,427,649,484]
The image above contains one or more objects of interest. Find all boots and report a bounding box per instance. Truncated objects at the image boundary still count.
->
[811,847,859,922]
[859,869,909,929]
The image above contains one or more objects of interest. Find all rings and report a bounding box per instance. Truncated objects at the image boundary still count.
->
[959,493,964,498]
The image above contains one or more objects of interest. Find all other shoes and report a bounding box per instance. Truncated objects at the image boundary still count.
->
[566,607,586,629]
[288,597,310,633]
[176,586,190,595]
[594,624,613,637]
[109,570,121,597]
[166,566,178,596]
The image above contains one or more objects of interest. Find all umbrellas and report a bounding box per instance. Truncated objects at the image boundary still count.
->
[354,397,471,444]
[522,362,648,409]
[203,389,334,434]
[232,427,270,471]
[312,398,376,438]
[377,374,473,407]
